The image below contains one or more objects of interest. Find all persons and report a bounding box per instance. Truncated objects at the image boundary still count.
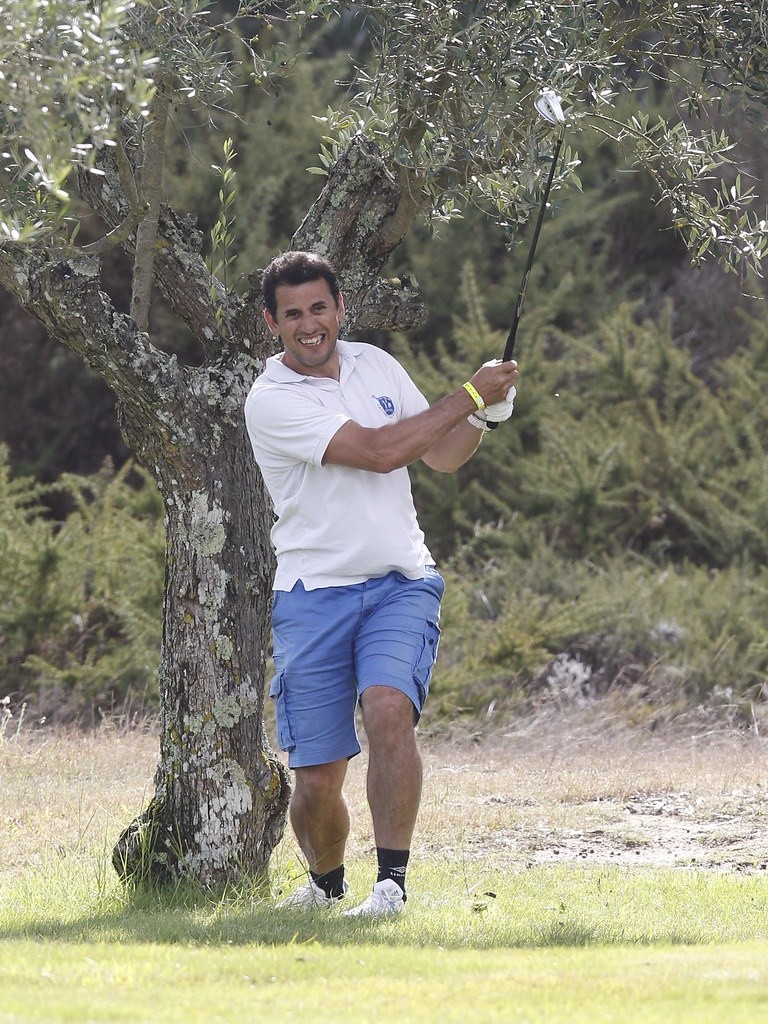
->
[243,249,519,917]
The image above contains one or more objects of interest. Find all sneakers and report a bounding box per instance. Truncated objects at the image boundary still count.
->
[275,877,349,910]
[340,878,407,919]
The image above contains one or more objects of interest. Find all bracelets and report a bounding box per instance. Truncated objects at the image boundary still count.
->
[462,382,485,410]
[465,413,492,432]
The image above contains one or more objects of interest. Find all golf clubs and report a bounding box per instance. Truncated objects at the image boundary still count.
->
[486,90,567,429]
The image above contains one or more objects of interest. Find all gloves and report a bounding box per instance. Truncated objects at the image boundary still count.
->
[473,359,516,422]
